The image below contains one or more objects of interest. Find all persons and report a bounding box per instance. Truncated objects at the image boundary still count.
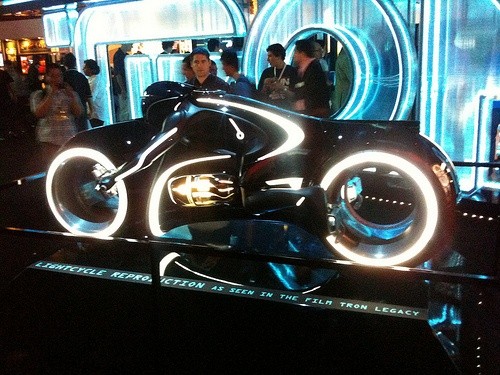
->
[188,47,230,93]
[310,40,332,111]
[208,60,217,76]
[63,53,93,133]
[182,56,196,85]
[220,50,257,100]
[30,63,84,174]
[82,59,105,128]
[161,41,175,55]
[113,44,133,122]
[0,58,45,187]
[207,38,220,52]
[266,39,332,119]
[331,47,353,116]
[257,43,297,107]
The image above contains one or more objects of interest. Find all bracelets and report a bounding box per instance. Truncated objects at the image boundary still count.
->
[282,88,288,94]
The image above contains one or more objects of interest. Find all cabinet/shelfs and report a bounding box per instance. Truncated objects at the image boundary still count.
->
[0,37,73,74]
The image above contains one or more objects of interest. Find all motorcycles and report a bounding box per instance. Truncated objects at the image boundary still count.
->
[44,81,461,268]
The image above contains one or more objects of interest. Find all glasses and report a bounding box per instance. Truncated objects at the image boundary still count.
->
[313,48,323,53]
